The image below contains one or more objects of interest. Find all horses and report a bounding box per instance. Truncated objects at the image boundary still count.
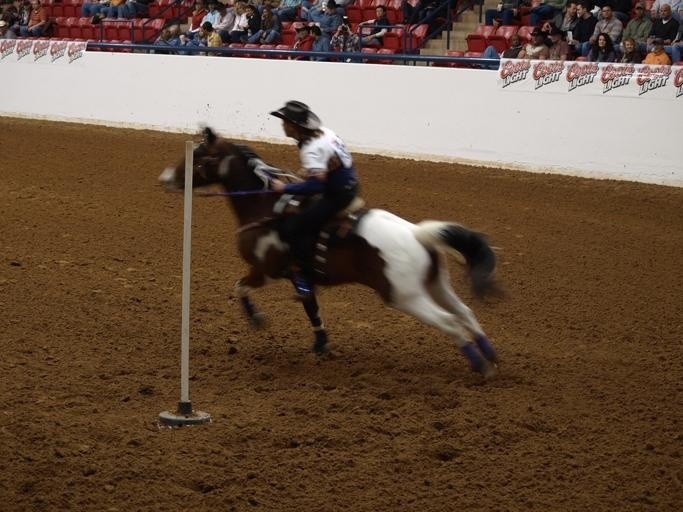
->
[157,127,500,383]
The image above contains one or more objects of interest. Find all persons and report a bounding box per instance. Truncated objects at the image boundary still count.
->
[269,99,360,303]
[154,0,393,64]
[483,0,683,65]
[0,0,49,40]
[81,0,149,19]
[404,0,450,33]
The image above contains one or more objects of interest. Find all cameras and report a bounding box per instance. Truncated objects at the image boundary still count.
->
[341,15,349,32]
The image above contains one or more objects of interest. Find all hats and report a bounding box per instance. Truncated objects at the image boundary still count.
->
[324,1,342,10]
[271,101,321,131]
[635,2,644,9]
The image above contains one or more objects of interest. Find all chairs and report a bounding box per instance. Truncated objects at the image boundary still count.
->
[0,0,536,70]
